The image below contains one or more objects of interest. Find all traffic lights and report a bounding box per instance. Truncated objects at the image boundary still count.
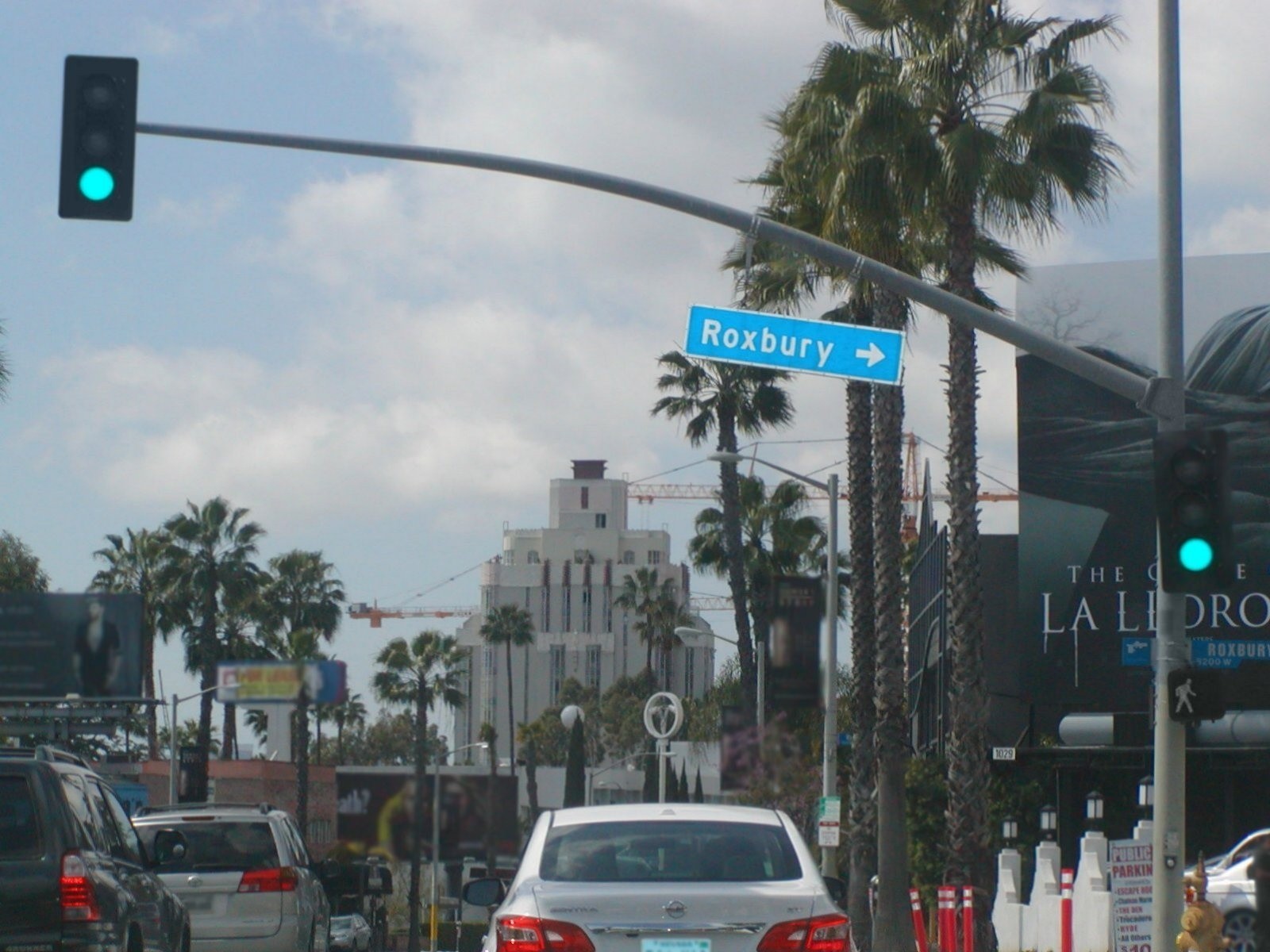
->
[1166,666,1228,723]
[1151,427,1235,595]
[57,55,139,222]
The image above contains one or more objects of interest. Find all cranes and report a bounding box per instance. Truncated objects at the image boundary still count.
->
[625,428,1019,543]
[346,552,503,629]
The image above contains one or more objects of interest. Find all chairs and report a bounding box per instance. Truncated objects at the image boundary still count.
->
[700,836,766,877]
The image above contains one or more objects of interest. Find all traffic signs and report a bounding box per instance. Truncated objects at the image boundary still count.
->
[682,303,907,388]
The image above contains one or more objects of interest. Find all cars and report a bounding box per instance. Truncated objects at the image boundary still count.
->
[1181,826,1270,952]
[461,798,857,952]
[327,911,374,952]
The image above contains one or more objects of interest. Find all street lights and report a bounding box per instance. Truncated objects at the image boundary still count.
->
[429,741,490,952]
[707,452,842,911]
[672,625,766,797]
[167,682,241,805]
[584,750,676,808]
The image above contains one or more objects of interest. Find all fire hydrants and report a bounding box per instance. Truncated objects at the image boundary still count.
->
[1175,902,1231,952]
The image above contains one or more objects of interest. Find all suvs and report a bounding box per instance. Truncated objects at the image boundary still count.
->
[123,799,341,952]
[0,741,195,951]
